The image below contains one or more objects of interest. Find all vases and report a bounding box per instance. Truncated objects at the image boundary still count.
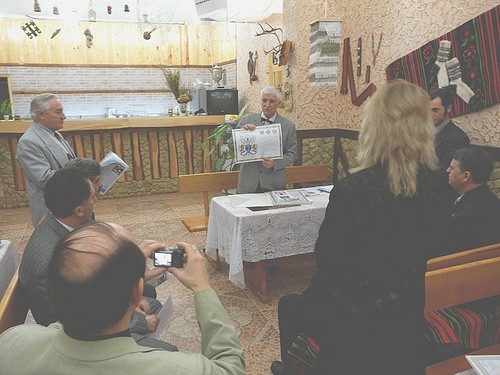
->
[179,103,187,116]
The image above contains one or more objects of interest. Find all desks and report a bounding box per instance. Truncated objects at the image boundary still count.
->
[205,184,334,303]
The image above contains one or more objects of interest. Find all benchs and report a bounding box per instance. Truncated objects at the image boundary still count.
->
[178,164,332,235]
[0,214,49,334]
[423,243,500,375]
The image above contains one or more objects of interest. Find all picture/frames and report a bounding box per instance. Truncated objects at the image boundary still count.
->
[0,74,15,121]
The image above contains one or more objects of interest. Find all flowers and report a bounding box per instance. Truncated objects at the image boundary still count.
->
[162,68,193,104]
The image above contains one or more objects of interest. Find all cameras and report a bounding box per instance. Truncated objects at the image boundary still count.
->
[152,247,184,268]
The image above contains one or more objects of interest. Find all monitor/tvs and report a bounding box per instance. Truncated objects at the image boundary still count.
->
[206,89,238,115]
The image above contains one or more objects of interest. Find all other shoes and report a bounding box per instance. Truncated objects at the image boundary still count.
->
[271,361,283,375]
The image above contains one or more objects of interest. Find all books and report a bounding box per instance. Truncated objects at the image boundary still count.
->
[98,151,129,196]
[453,354,500,375]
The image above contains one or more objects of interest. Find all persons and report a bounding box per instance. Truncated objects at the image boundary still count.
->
[16,93,77,230]
[428,87,469,208]
[271,80,460,375]
[447,145,499,251]
[0,221,247,375]
[17,158,178,353]
[229,87,298,194]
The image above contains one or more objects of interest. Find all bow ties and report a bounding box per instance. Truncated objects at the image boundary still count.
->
[261,117,273,124]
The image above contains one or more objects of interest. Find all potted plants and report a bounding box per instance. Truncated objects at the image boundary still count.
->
[0,100,12,120]
